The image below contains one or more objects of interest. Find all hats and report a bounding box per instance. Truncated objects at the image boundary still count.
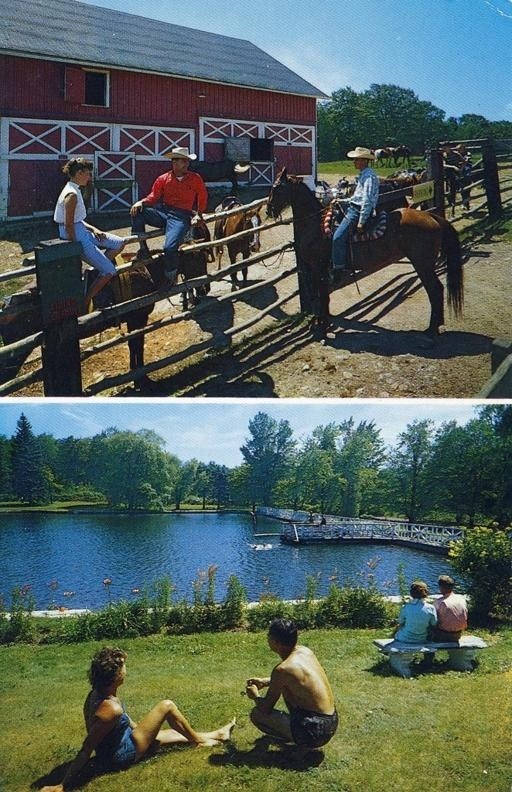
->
[347,147,374,159]
[164,147,197,161]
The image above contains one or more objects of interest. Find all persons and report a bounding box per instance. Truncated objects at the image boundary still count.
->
[439,140,473,209]
[419,575,468,665]
[378,581,438,657]
[129,147,208,290]
[316,514,326,527]
[328,147,380,291]
[38,645,236,792]
[53,158,125,316]
[245,618,338,761]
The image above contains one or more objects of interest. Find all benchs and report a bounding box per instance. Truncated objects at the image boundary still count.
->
[373,634,489,678]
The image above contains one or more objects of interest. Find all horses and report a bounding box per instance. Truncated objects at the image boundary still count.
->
[371,145,411,168]
[182,210,212,313]
[213,195,263,292]
[0,245,211,393]
[337,169,427,198]
[265,166,465,350]
